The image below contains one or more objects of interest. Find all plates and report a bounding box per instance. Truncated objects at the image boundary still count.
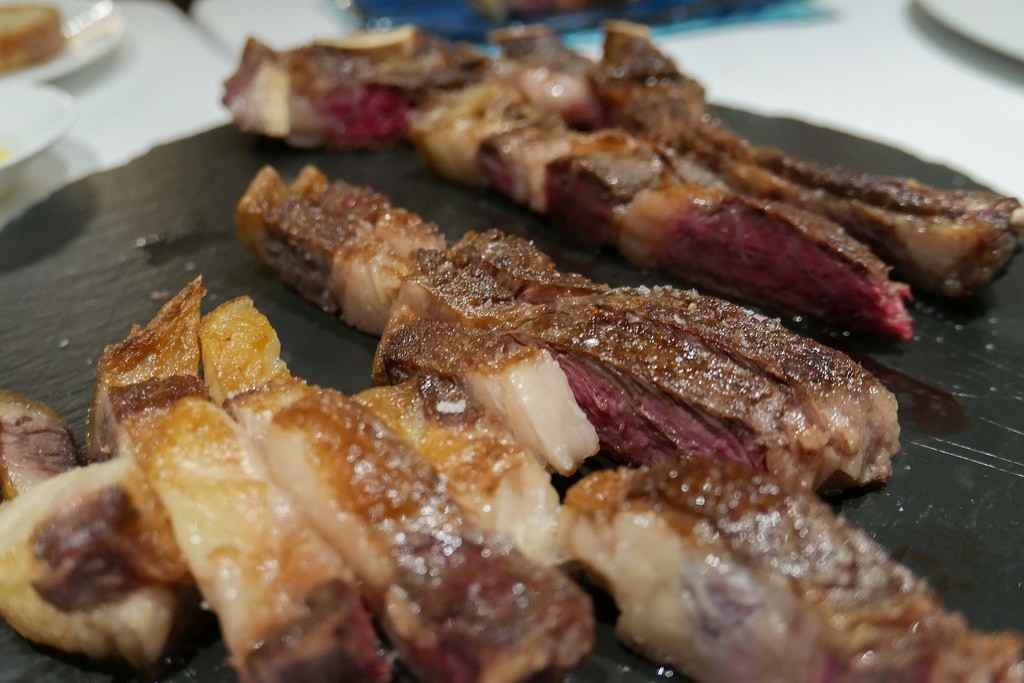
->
[919,0,1024,66]
[0,0,122,83]
[0,79,76,196]
